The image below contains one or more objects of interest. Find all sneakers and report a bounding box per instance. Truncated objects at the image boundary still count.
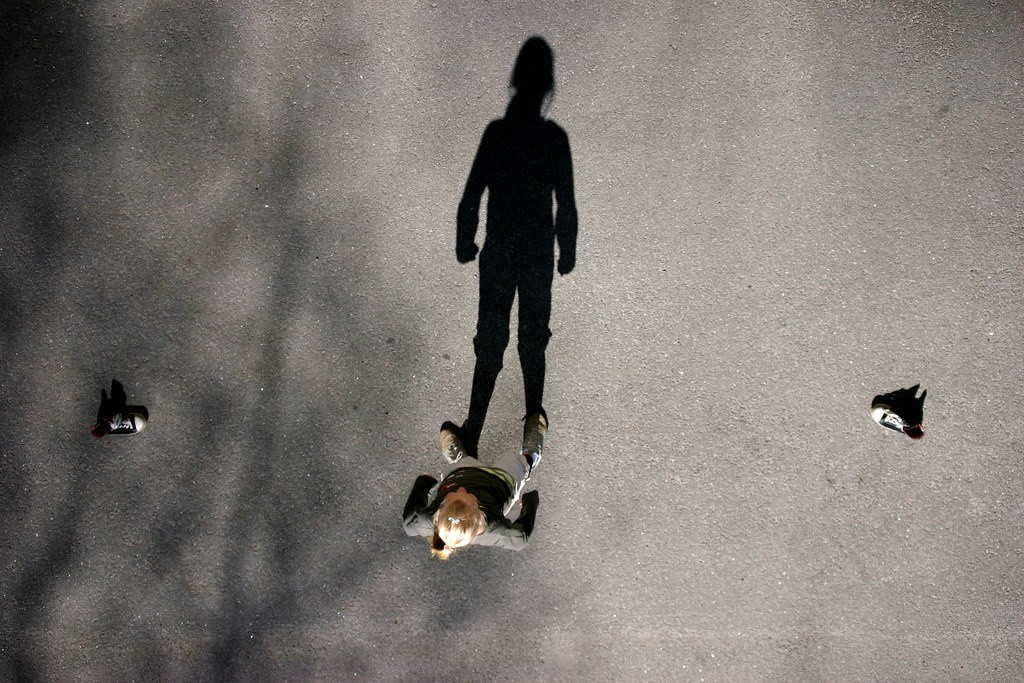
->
[521,414,547,471]
[439,428,468,465]
[870,403,925,439]
[91,409,146,438]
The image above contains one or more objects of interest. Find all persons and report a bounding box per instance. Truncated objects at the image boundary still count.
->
[401,412,547,560]
